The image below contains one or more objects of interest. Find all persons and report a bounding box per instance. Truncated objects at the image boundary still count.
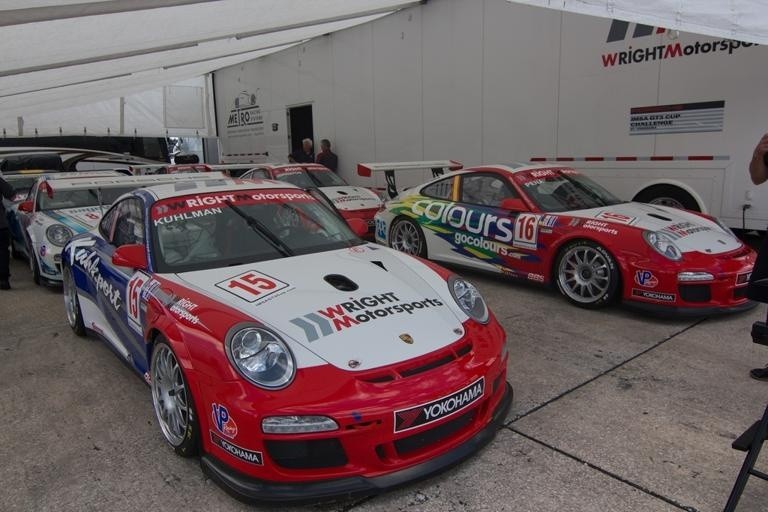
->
[316,138,338,174]
[1,172,18,292]
[286,138,315,165]
[747,133,768,187]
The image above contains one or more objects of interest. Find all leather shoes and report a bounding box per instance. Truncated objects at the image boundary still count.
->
[748,367,768,382]
